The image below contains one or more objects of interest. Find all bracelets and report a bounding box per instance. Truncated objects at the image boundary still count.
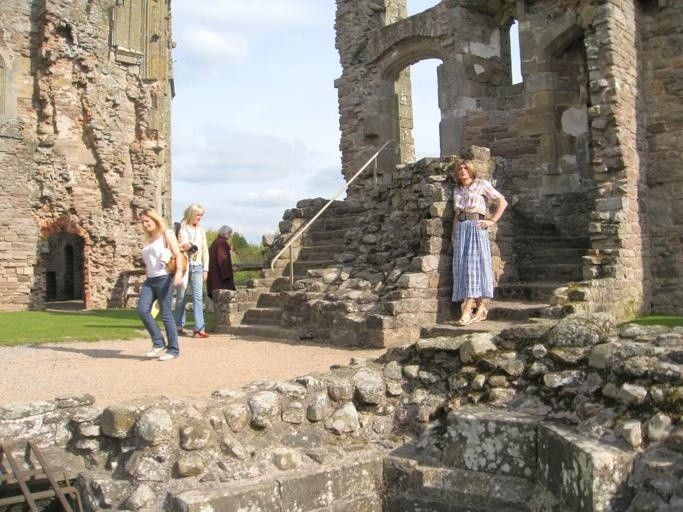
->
[490,217,497,223]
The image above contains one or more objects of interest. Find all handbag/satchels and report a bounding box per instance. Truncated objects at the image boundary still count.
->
[163,229,188,273]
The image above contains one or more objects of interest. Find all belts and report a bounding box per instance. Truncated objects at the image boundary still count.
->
[456,212,484,221]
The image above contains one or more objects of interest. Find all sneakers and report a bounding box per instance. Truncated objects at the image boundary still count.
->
[146,346,166,357]
[159,353,173,361]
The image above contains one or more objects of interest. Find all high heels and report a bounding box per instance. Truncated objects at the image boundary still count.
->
[194,330,208,337]
[177,329,183,333]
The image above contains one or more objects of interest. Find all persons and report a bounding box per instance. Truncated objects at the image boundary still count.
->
[206,224,237,303]
[171,202,209,338]
[138,208,183,360]
[450,160,509,325]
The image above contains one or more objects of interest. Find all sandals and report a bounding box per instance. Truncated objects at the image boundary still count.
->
[457,307,488,326]
